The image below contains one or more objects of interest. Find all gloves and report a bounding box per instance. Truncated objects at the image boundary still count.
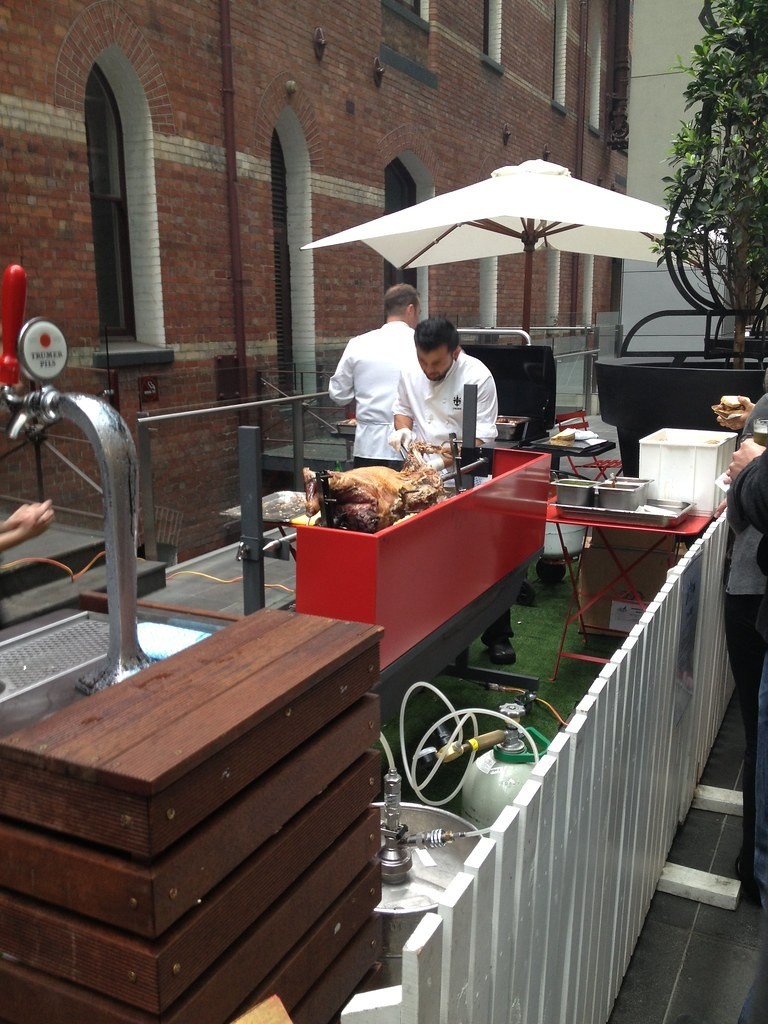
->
[427,458,445,473]
[388,427,412,452]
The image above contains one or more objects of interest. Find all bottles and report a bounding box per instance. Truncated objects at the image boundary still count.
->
[332,460,343,472]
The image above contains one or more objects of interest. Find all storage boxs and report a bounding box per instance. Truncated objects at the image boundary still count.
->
[495,415,531,441]
[590,528,679,552]
[638,427,738,517]
[577,536,688,638]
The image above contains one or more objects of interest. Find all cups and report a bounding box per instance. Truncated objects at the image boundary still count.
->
[752,418,768,448]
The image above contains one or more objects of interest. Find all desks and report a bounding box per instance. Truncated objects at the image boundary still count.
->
[546,495,711,682]
[523,442,617,474]
[329,426,527,459]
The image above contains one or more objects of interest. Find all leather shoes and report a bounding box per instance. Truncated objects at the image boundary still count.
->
[490,639,515,666]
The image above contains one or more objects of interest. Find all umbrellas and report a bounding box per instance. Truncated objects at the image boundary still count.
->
[300,157,735,347]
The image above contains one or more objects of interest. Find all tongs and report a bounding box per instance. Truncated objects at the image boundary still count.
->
[398,443,411,462]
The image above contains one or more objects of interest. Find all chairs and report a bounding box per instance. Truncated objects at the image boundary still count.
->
[556,410,622,481]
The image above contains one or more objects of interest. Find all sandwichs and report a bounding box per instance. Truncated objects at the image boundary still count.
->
[717,395,752,414]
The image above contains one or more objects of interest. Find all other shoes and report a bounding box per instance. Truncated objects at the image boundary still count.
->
[735,855,744,876]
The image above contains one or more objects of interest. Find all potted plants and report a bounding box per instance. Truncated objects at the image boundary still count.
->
[594,0,768,477]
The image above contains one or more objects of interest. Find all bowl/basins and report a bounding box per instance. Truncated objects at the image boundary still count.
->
[333,418,356,435]
[496,414,529,441]
[592,482,643,512]
[607,475,654,506]
[550,478,599,507]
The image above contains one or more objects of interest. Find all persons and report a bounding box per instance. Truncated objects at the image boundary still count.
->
[392,320,519,667]
[711,361,768,1024]
[325,284,421,469]
[0,496,60,550]
